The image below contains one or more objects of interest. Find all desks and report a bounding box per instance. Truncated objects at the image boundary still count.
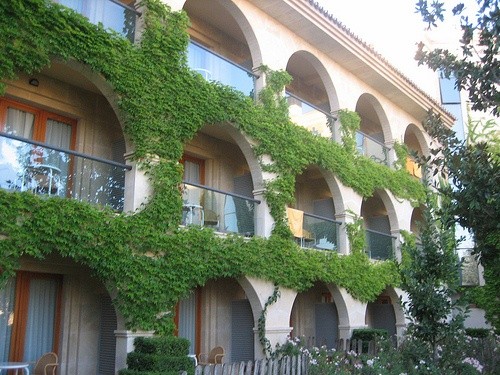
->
[181,204,206,227]
[190,65,214,84]
[187,355,199,367]
[25,164,62,199]
[0,361,32,375]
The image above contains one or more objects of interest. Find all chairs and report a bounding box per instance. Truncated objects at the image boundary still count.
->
[26,352,59,375]
[199,208,219,228]
[198,346,224,364]
[302,228,318,248]
